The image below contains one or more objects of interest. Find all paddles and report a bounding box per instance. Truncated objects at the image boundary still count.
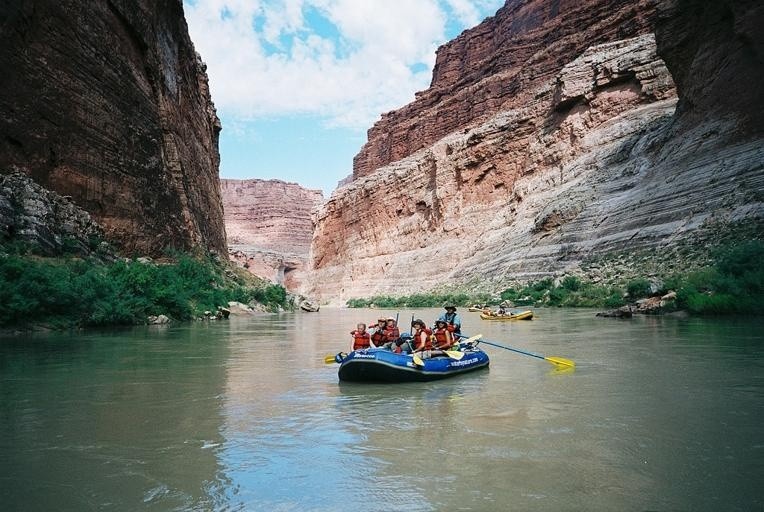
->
[533,315,540,319]
[436,346,464,361]
[326,354,348,364]
[406,338,425,367]
[453,332,576,369]
[454,333,483,347]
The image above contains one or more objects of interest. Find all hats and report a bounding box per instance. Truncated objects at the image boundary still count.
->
[435,318,447,326]
[411,319,424,327]
[444,303,456,311]
[378,317,396,322]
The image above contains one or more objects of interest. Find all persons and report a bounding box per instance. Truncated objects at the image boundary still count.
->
[496,304,513,316]
[408,318,432,359]
[385,318,400,349]
[437,302,462,344]
[430,316,455,357]
[350,323,377,352]
[368,318,390,347]
[483,305,500,316]
[474,303,490,309]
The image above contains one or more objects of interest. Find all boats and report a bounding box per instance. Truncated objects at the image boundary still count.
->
[468,307,533,321]
[338,343,489,383]
